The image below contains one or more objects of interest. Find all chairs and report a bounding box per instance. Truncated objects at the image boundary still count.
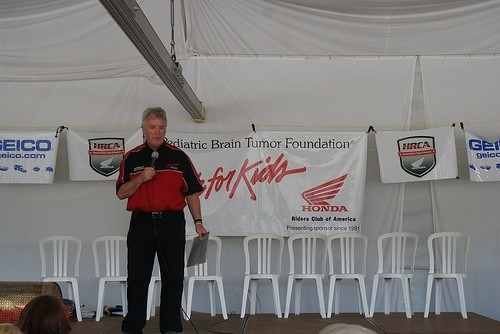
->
[284,232,329,319]
[181,235,228,321]
[326,232,371,318]
[424,231,471,319]
[369,231,419,319]
[240,233,285,319]
[146,256,187,319]
[38,235,83,322]
[92,236,128,322]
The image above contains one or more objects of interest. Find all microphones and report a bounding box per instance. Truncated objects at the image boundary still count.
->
[150,151,159,167]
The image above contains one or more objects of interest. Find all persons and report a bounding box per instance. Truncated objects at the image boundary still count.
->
[116,107,214,334]
[19,295,71,334]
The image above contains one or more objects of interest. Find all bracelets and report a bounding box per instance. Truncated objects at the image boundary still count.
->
[196,222,201,225]
[194,218,202,224]
